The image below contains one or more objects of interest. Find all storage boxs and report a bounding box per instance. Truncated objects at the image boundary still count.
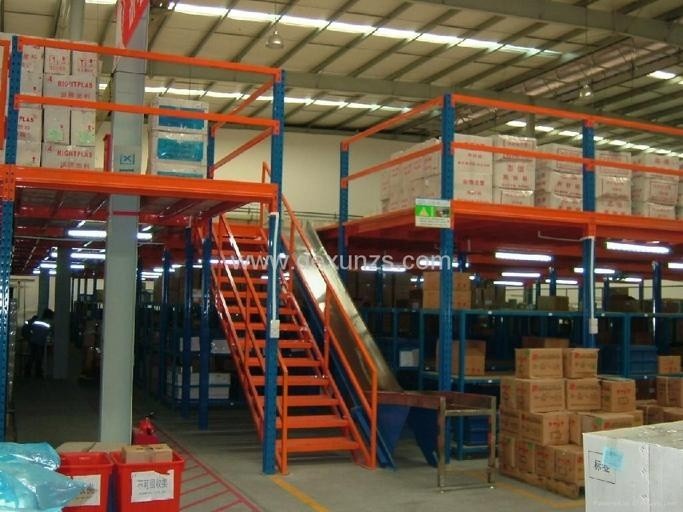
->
[0,29,209,179]
[55,441,185,511]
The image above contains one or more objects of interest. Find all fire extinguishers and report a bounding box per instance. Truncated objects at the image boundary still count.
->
[134,411,159,444]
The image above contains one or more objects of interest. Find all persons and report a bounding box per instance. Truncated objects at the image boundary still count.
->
[20,315,37,338]
[22,308,54,380]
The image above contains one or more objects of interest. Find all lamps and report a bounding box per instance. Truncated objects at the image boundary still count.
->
[264,1,285,49]
[578,9,594,99]
